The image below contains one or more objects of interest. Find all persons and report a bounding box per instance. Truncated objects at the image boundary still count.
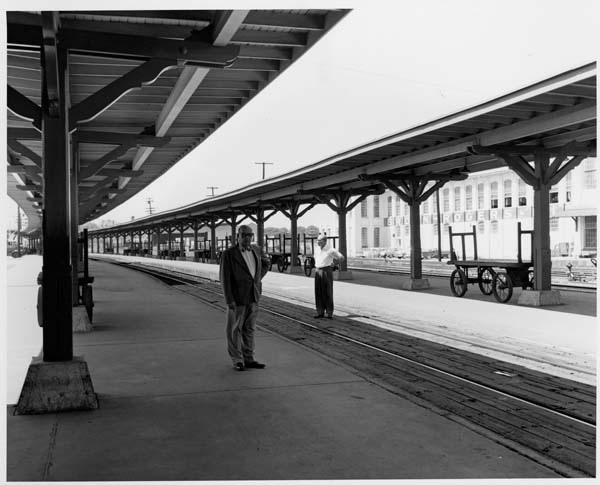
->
[313,234,345,318]
[219,225,271,371]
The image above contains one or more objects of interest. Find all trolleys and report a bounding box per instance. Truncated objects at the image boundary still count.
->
[447,222,534,303]
[124,233,339,277]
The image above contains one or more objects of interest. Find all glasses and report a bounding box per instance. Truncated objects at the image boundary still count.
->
[240,231,254,237]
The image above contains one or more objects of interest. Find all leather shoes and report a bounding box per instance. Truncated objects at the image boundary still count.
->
[245,361,266,369]
[234,363,246,372]
[328,316,334,319]
[311,313,325,319]
